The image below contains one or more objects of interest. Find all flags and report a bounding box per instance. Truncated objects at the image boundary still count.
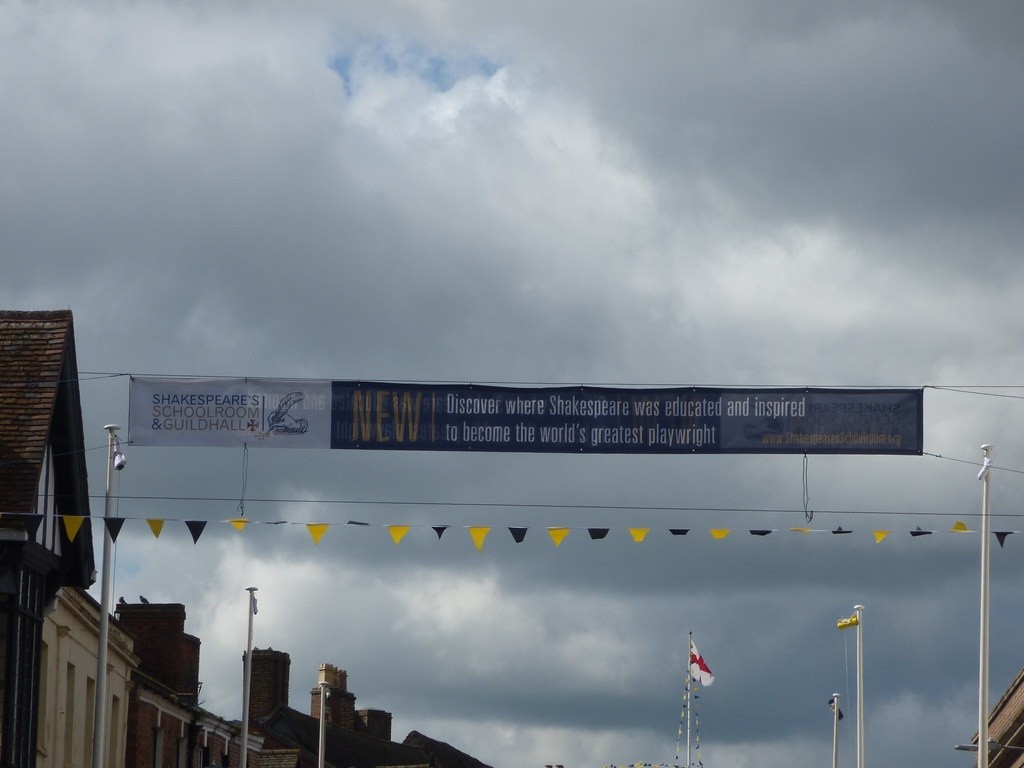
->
[837,611,858,629]
[687,638,715,686]
